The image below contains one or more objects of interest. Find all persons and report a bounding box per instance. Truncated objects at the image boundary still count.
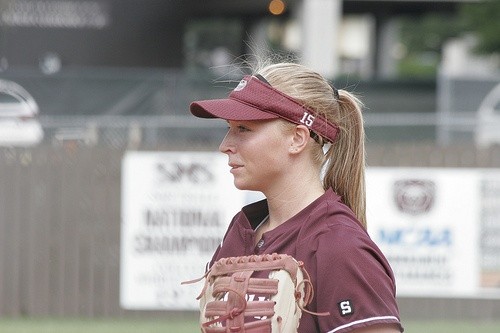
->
[196,60,404,333]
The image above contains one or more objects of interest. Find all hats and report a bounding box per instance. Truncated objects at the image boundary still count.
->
[190,75,340,143]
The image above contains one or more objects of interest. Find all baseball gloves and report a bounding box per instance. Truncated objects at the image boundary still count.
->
[194,255,315,333]
